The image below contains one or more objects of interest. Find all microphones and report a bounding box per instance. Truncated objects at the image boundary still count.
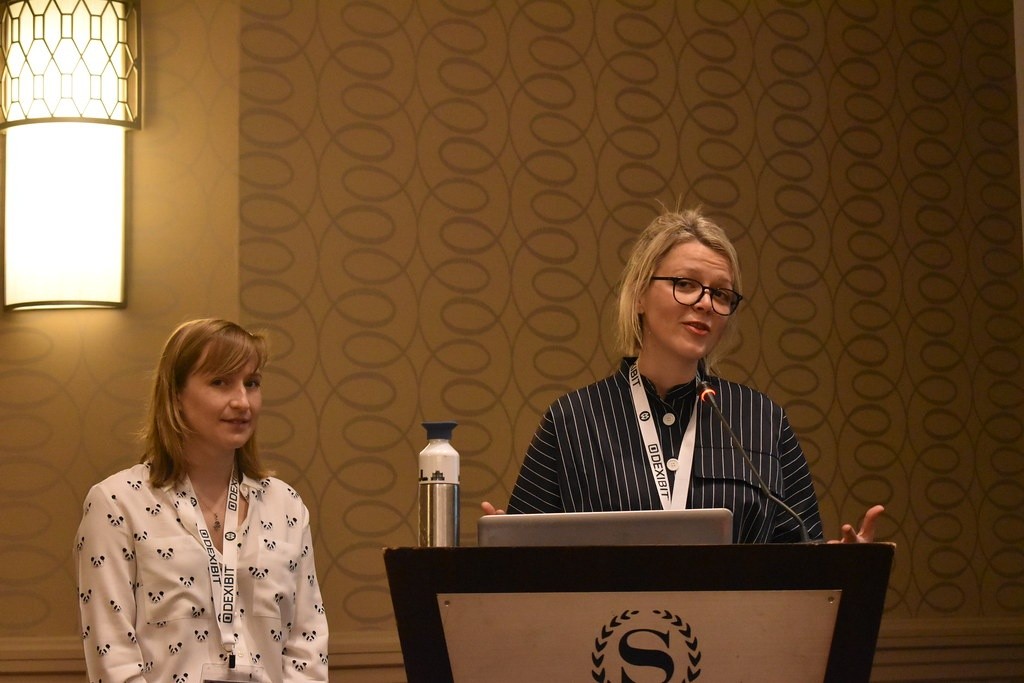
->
[696,380,811,542]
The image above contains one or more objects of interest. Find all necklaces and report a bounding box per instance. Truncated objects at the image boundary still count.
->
[194,490,228,531]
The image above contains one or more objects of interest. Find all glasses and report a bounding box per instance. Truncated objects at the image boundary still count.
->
[650,276,744,316]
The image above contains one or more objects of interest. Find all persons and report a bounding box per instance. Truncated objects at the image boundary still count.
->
[75,319,328,683]
[481,208,883,542]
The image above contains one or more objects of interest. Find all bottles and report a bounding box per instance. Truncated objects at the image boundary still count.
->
[418,421,461,548]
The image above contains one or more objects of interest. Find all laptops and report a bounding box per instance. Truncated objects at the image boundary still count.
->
[477,508,733,547]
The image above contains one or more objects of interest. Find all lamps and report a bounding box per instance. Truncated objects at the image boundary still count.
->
[2,1,147,312]
